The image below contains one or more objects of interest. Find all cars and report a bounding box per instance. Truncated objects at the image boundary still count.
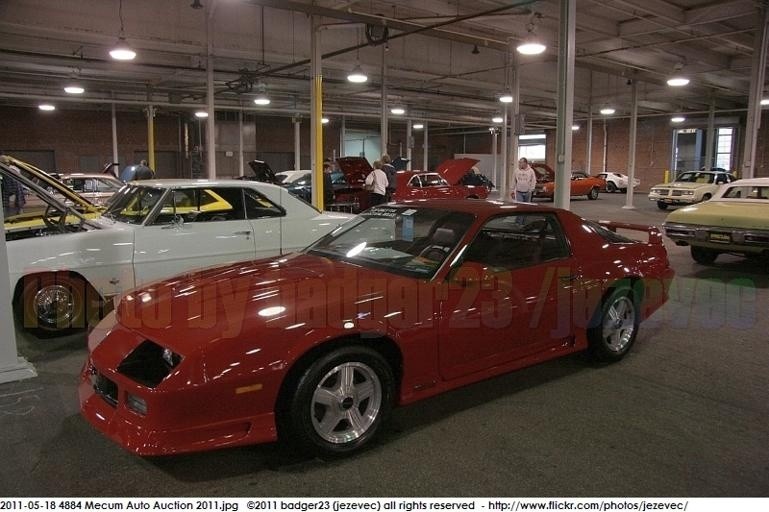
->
[528,161,607,203]
[0,160,366,340]
[648,171,736,210]
[683,167,736,183]
[242,153,495,209]
[0,155,272,231]
[662,176,769,265]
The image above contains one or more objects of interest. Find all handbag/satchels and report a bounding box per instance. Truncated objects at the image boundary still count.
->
[365,171,375,191]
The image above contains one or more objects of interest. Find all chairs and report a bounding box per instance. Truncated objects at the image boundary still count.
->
[419,228,456,265]
[205,213,232,221]
[185,209,207,221]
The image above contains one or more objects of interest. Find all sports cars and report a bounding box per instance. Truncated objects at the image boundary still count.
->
[593,171,640,193]
[77,198,675,458]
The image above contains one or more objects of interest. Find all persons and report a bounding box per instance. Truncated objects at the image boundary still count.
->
[0,156,25,217]
[361,160,389,204]
[509,158,538,226]
[131,160,152,179]
[382,155,397,202]
[323,163,336,210]
[323,158,331,164]
